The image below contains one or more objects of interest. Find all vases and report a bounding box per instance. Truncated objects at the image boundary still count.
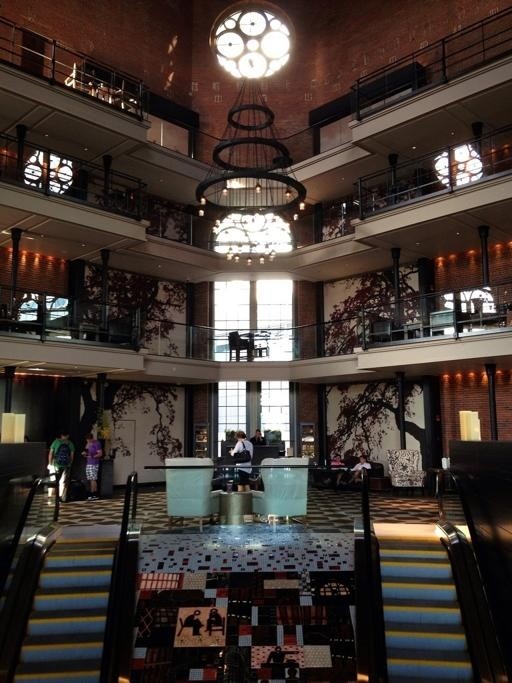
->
[98,438,111,459]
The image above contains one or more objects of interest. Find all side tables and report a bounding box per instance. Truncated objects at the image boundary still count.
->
[368,477,390,494]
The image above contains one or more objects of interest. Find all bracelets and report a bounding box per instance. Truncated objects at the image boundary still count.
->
[91,455,94,458]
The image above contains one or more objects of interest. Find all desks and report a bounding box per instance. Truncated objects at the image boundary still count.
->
[401,321,424,340]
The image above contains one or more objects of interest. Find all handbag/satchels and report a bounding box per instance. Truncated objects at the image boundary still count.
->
[234,443,251,464]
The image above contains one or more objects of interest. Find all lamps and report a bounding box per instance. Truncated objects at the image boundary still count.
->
[195,2,308,265]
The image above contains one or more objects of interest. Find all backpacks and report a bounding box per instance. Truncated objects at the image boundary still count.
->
[55,442,71,467]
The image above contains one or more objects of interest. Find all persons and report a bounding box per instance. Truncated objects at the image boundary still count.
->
[327,454,345,486]
[44,431,75,502]
[348,454,372,484]
[227,430,253,493]
[249,429,266,446]
[79,433,103,503]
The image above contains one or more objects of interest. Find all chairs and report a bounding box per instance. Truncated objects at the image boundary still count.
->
[387,448,426,497]
[228,331,255,361]
[165,456,308,531]
[370,322,392,343]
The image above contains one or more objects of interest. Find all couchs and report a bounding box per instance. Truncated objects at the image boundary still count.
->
[308,456,384,492]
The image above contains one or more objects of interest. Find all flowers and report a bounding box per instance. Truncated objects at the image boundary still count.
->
[96,411,111,440]
